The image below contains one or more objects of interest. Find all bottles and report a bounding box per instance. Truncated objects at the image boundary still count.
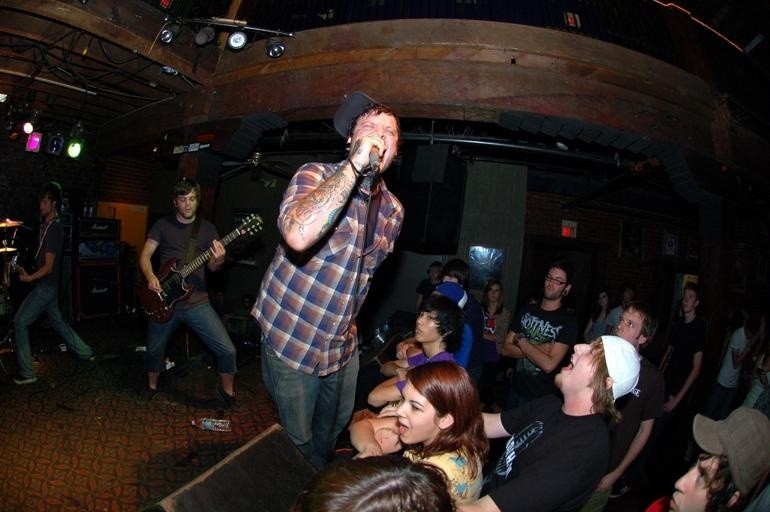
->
[82,201,95,219]
[191,418,232,432]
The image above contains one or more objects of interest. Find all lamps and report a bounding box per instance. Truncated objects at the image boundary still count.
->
[4,105,92,160]
[160,17,295,58]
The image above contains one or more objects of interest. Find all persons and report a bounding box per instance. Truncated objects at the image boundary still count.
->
[12,181,96,385]
[293,259,770,512]
[252,91,404,470]
[135,176,244,414]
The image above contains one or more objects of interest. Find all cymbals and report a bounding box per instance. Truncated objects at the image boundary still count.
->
[0,219,22,227]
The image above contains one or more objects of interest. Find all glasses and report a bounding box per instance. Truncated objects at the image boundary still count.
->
[545,275,569,286]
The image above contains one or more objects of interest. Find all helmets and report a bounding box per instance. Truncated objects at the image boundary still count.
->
[600,335,640,405]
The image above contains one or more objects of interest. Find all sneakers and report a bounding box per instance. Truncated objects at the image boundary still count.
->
[216,384,246,415]
[136,386,158,405]
[87,354,106,377]
[14,374,39,385]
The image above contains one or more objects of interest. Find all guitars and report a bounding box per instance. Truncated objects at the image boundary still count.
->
[139,214,264,323]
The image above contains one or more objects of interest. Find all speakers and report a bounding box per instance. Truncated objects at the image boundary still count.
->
[395,144,467,255]
[143,424,321,512]
[75,216,121,263]
[70,264,122,321]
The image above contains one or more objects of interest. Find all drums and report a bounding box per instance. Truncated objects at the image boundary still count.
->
[1,246,17,273]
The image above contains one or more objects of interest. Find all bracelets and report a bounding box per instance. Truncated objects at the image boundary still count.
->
[347,158,361,175]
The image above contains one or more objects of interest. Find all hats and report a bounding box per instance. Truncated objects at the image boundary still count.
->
[693,405,769,494]
[334,91,401,139]
[435,282,468,310]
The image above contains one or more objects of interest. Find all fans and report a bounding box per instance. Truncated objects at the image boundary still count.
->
[219,151,296,182]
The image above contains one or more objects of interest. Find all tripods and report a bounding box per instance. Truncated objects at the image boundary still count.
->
[0,254,41,360]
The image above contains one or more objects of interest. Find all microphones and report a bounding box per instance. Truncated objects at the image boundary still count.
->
[369,147,380,177]
